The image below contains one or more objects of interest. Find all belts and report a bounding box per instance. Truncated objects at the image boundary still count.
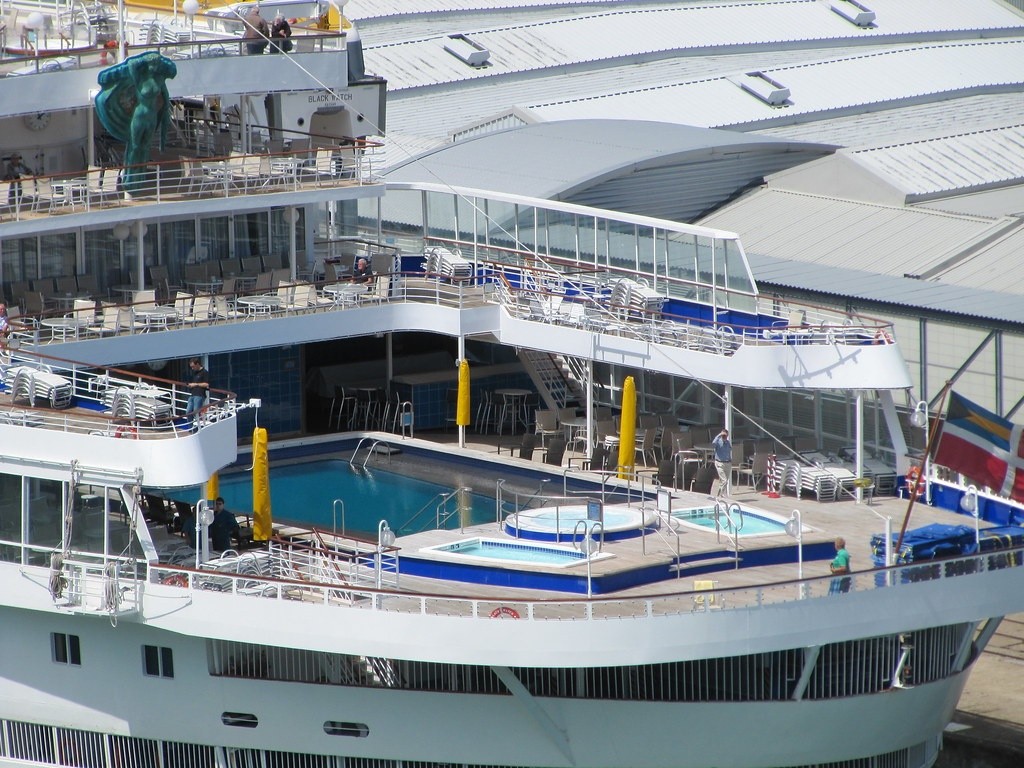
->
[715,459,731,463]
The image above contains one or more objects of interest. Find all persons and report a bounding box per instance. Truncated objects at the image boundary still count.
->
[183,507,200,549]
[187,359,210,430]
[712,429,732,497]
[351,259,372,285]
[271,15,291,53]
[208,497,239,551]
[6,152,32,204]
[124,54,178,187]
[242,7,269,55]
[0,304,9,354]
[829,537,850,594]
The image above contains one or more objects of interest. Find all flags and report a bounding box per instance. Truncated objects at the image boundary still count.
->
[933,390,1024,506]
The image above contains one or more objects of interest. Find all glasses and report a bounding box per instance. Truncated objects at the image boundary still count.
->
[216,503,223,505]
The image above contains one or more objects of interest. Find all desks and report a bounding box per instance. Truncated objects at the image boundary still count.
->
[614,427,661,461]
[695,443,715,468]
[202,162,242,194]
[41,317,88,344]
[48,291,92,317]
[659,425,688,432]
[323,283,369,309]
[132,307,178,333]
[237,295,282,322]
[52,179,90,211]
[235,276,258,296]
[560,416,596,450]
[349,384,386,428]
[80,494,98,508]
[496,388,533,435]
[270,157,305,191]
[316,264,349,280]
[187,281,224,296]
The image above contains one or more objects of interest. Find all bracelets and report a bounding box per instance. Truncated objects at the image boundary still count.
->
[196,383,199,386]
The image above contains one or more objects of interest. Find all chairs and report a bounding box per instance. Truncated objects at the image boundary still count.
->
[6,364,175,430]
[0,130,392,339]
[166,545,298,596]
[328,383,405,432]
[117,488,193,541]
[446,388,897,504]
[421,244,810,354]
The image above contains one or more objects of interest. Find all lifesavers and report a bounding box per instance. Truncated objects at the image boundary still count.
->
[489,607,518,618]
[905,465,923,498]
[162,576,188,586]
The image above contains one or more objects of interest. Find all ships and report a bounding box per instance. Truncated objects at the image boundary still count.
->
[0,0,1024,768]
